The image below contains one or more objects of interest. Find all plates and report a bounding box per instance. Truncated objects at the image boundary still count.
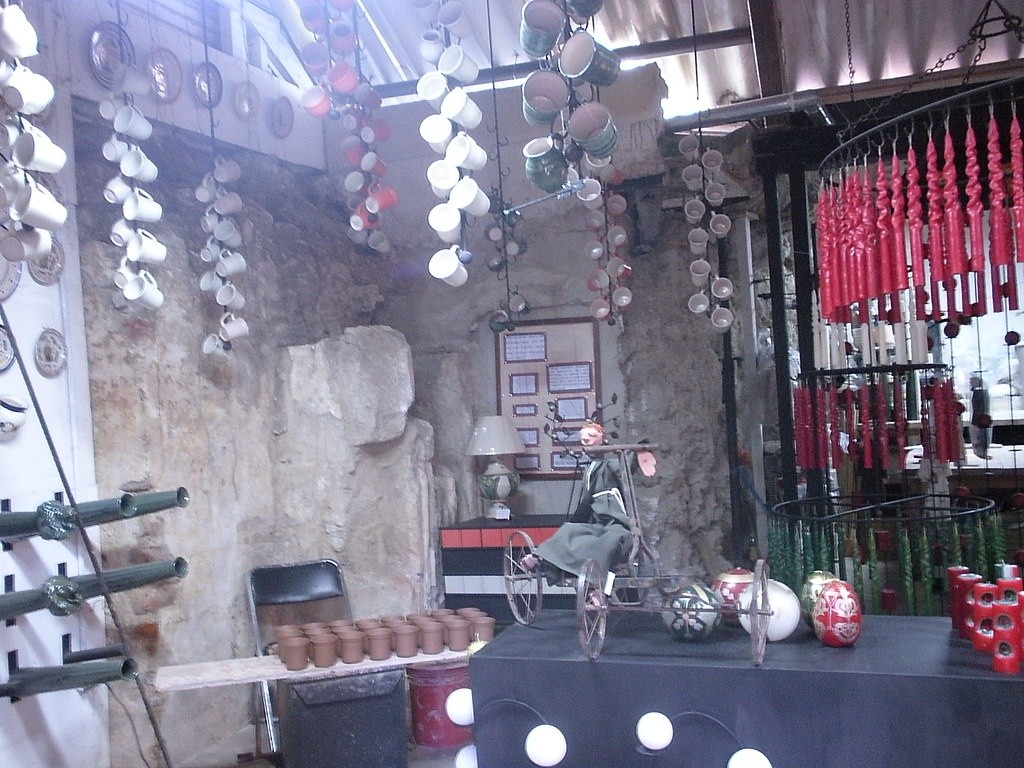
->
[193,62,222,108]
[89,21,136,90]
[29,236,65,283]
[0,324,16,371]
[35,329,67,375]
[0,224,22,301]
[147,48,183,103]
[235,81,261,121]
[271,97,294,138]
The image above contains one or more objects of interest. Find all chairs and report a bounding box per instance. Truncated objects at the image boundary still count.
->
[247,559,352,753]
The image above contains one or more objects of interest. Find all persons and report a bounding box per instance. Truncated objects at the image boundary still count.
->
[521,423,657,587]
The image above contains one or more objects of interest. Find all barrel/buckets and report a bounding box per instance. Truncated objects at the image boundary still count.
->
[407,668,475,748]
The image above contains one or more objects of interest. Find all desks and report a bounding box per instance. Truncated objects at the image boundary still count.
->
[904,445,1024,504]
[442,513,581,623]
[470,607,1024,768]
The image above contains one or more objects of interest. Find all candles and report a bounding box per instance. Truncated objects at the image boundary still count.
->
[765,99,1024,675]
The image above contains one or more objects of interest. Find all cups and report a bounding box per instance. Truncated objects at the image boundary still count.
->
[195,155,247,358]
[0,0,67,262]
[273,608,495,670]
[299,0,396,254]
[410,0,733,332]
[99,65,166,310]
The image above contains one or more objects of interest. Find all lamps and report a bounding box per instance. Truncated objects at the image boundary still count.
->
[467,416,527,522]
